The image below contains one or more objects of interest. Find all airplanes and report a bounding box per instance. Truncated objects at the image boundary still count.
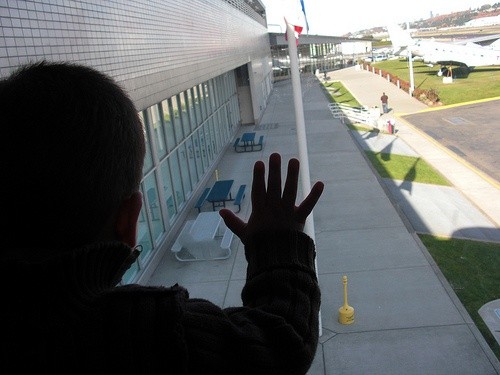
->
[412,38,500,77]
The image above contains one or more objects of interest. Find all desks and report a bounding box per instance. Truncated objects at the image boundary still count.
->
[241,133,255,151]
[207,180,234,211]
[189,212,222,242]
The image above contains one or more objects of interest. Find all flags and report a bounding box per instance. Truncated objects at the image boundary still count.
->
[284,0,309,46]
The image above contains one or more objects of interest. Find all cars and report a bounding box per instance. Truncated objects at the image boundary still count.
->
[373,47,405,61]
[345,54,373,64]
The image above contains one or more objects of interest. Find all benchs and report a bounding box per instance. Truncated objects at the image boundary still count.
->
[194,185,246,214]
[233,136,263,153]
[171,220,234,262]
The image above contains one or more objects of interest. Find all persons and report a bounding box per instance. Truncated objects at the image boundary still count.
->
[0,59,325,375]
[381,92,388,113]
